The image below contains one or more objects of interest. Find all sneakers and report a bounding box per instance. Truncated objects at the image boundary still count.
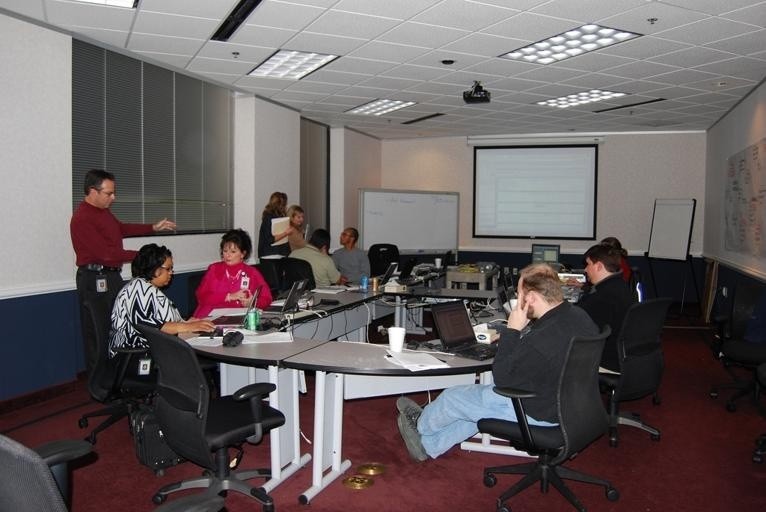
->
[395,395,422,421]
[396,414,428,462]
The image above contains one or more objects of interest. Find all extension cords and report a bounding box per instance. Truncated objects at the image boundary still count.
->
[415,273,432,281]
[283,310,312,320]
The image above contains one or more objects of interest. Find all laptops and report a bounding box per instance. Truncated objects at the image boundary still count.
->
[209,287,261,327]
[431,300,501,361]
[262,278,309,312]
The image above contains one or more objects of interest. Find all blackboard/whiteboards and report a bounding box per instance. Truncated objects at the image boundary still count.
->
[358,188,460,255]
[646,197,697,263]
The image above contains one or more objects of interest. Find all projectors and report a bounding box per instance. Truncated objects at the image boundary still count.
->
[463,81,491,103]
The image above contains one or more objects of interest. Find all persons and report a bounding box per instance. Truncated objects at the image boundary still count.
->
[331,228,371,283]
[575,245,633,370]
[602,236,634,282]
[289,229,347,286]
[71,169,176,378]
[107,243,215,381]
[259,192,291,287]
[184,228,273,321]
[396,263,601,464]
[287,206,306,251]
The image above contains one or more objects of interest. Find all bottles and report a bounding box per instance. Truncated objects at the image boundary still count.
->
[303,224,309,240]
[361,272,368,290]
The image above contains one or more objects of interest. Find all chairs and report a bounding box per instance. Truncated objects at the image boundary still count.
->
[707,314,766,418]
[0,434,228,510]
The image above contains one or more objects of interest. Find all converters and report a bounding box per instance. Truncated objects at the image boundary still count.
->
[256,321,273,331]
[321,299,339,305]
[416,271,428,275]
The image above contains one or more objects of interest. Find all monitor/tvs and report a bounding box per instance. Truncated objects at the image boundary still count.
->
[531,244,560,273]
[380,262,399,285]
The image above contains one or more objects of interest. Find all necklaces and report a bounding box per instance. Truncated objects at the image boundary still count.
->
[226,269,242,286]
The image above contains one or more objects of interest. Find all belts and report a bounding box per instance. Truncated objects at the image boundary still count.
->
[78,262,121,272]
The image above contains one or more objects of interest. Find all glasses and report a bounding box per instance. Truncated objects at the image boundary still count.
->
[159,265,174,271]
[93,187,116,195]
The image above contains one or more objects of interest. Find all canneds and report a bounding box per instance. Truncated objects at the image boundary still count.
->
[248,311,257,331]
[251,309,259,324]
[360,275,368,290]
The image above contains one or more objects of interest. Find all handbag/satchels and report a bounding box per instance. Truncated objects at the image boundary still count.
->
[132,403,188,468]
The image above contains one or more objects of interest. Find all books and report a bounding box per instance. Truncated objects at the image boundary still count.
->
[310,285,350,295]
[269,216,290,246]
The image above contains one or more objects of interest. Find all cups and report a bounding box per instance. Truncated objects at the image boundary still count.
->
[387,327,406,353]
[435,259,442,268]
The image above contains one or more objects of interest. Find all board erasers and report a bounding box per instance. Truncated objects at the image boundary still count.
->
[418,250,424,252]
